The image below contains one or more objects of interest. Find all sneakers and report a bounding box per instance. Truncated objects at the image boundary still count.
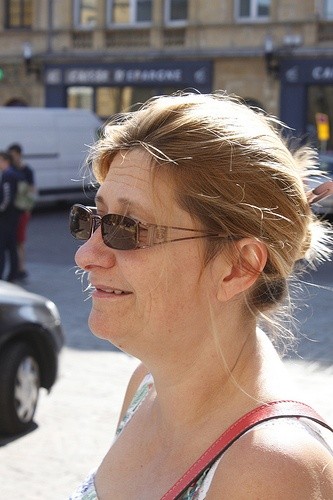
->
[9,270,28,281]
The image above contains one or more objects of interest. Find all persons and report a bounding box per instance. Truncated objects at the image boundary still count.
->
[0,144,37,282]
[68,91,333,500]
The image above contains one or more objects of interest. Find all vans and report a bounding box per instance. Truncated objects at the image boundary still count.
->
[0,106,104,208]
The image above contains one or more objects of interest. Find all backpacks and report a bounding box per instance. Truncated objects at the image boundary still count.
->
[15,181,37,209]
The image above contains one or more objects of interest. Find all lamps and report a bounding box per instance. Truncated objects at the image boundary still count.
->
[262,31,304,81]
[21,40,42,85]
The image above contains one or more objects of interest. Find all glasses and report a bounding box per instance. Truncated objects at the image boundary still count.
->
[68,204,225,251]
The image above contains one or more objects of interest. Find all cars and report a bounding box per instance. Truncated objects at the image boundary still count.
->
[0,281,65,436]
